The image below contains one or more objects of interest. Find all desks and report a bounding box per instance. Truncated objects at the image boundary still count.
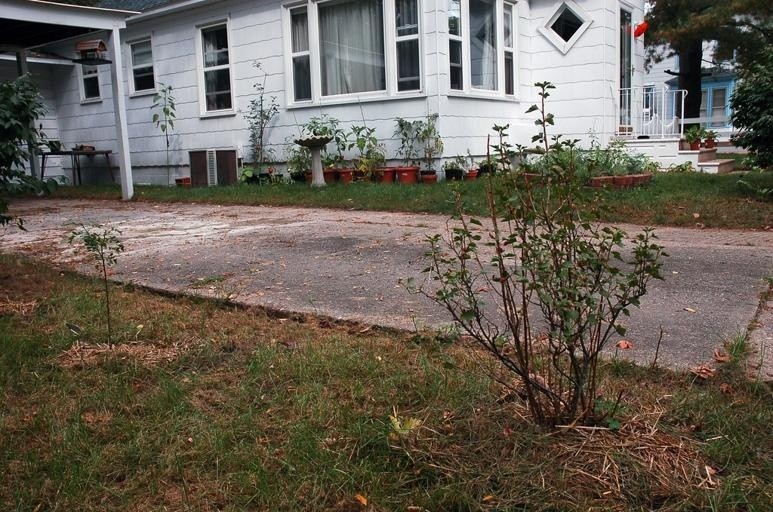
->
[35,150,116,187]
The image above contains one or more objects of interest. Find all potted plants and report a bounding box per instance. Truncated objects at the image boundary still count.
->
[442,140,659,189]
[685,124,721,150]
[239,63,439,188]
